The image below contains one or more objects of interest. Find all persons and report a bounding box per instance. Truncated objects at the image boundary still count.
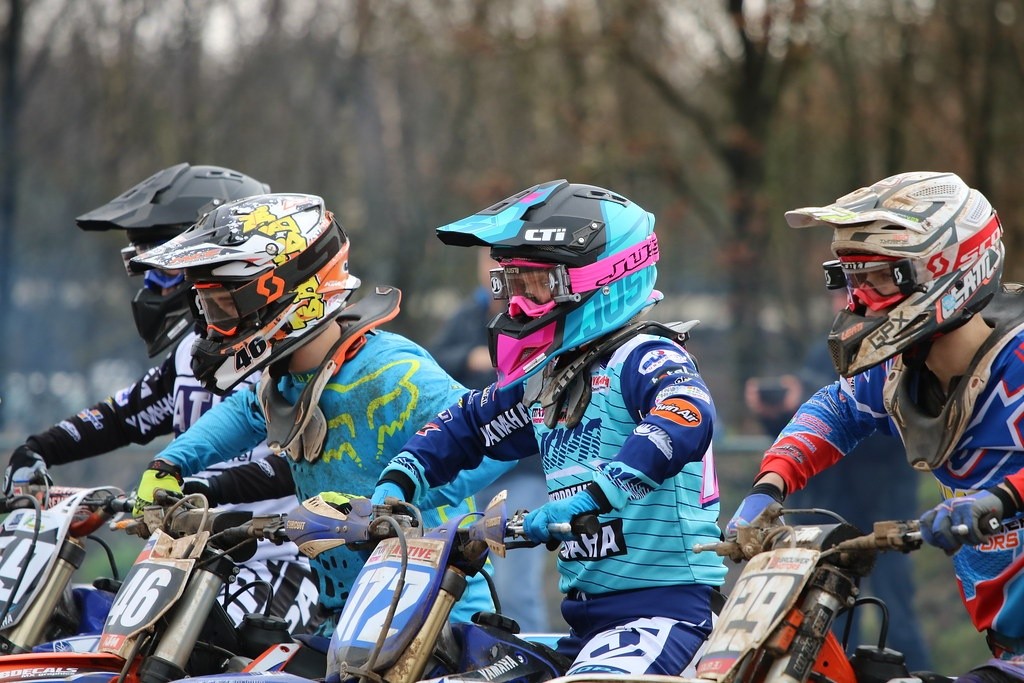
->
[0,162,318,683]
[755,301,933,674]
[347,177,728,683]
[723,169,1024,683]
[426,246,548,633]
[125,193,518,683]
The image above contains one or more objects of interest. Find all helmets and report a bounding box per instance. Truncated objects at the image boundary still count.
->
[77,161,271,358]
[785,170,1006,380]
[128,193,361,396]
[433,180,667,392]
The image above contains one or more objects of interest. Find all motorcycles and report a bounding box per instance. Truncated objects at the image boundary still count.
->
[0,450,1002,682]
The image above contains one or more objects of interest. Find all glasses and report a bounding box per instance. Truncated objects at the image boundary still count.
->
[842,259,916,313]
[499,260,570,318]
[193,283,242,336]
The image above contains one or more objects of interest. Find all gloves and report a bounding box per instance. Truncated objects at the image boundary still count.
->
[1,443,52,499]
[369,481,405,518]
[919,486,1019,556]
[132,460,185,518]
[522,483,606,552]
[726,483,787,564]
[183,475,216,510]
